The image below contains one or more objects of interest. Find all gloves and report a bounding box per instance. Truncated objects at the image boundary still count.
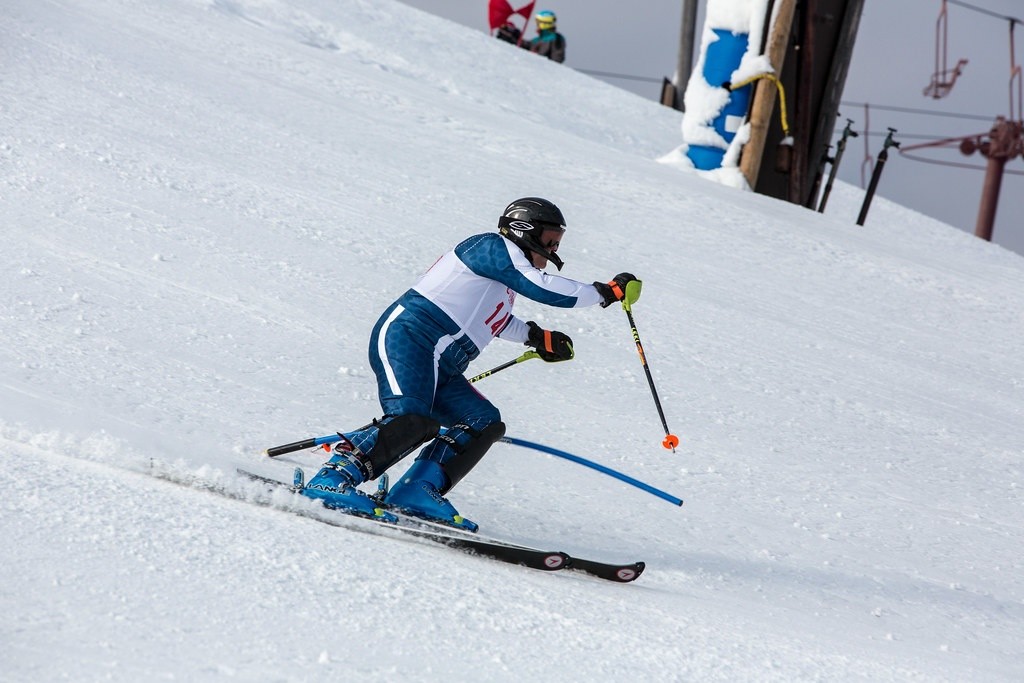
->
[524,320,573,362]
[593,272,637,308]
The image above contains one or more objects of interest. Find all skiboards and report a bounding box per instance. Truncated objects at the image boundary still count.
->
[233,465,645,584]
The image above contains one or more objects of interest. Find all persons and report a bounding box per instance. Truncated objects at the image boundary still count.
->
[514,10,566,64]
[304,197,641,532]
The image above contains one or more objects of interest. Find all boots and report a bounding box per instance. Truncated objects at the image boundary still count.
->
[293,452,400,523]
[375,457,480,532]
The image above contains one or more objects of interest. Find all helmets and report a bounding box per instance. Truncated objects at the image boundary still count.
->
[536,10,558,30]
[498,197,567,272]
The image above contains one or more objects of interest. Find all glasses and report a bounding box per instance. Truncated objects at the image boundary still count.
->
[528,221,566,252]
